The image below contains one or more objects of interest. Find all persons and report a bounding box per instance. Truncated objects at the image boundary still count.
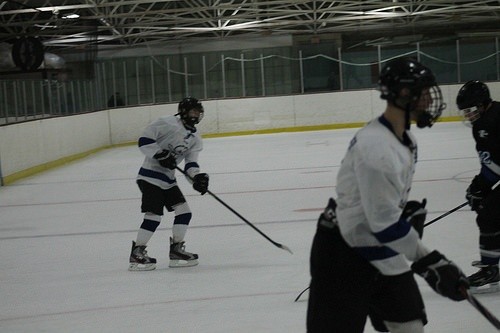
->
[456,78,500,288]
[130,97,209,264]
[306,57,472,333]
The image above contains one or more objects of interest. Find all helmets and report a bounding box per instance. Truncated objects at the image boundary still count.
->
[379,59,447,129]
[455,79,493,124]
[178,96,204,133]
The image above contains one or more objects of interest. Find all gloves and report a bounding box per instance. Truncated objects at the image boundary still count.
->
[410,250,473,301]
[402,198,427,240]
[153,149,177,170]
[192,173,209,195]
[466,174,493,213]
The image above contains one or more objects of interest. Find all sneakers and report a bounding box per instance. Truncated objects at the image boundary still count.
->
[128,241,157,271]
[466,261,500,294]
[169,236,199,267]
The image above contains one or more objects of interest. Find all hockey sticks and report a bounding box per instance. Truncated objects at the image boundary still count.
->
[293,181,500,303]
[175,165,295,256]
[467,291,500,333]
